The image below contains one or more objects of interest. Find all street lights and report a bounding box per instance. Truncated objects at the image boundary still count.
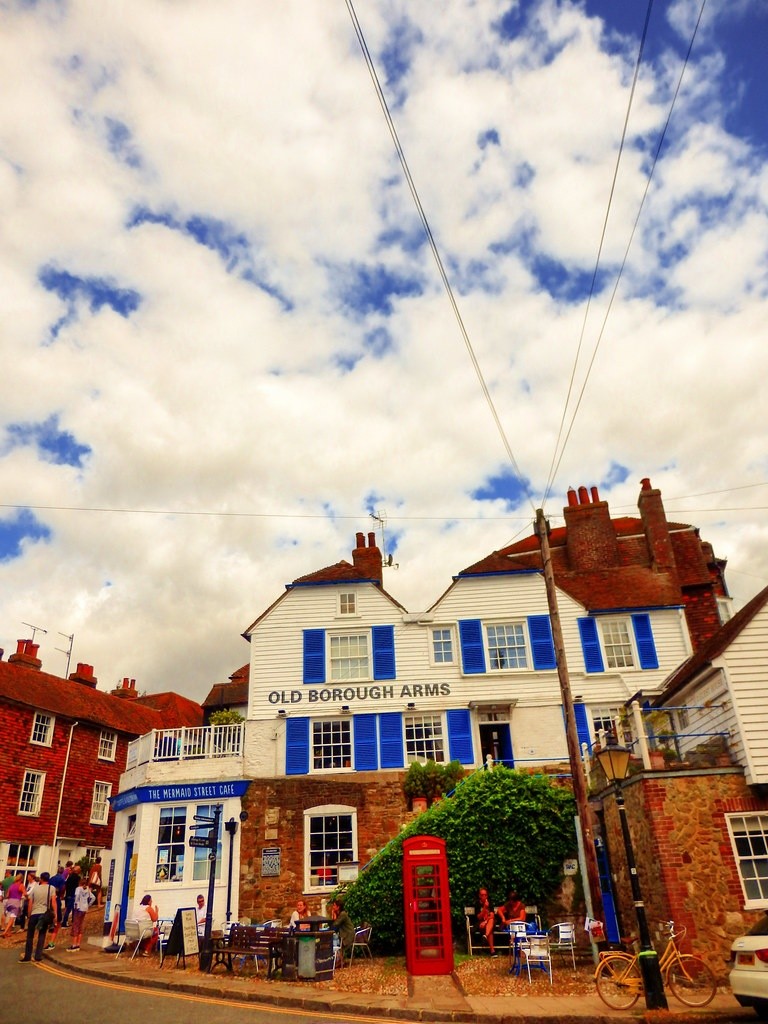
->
[594,725,676,1010]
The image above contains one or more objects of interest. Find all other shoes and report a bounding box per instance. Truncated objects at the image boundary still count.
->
[142,952,150,956]
[482,935,488,943]
[60,922,72,929]
[18,959,31,964]
[31,957,42,961]
[16,929,25,934]
[0,932,11,938]
[489,950,498,957]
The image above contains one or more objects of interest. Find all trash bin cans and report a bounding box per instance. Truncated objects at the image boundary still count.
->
[293,915,335,982]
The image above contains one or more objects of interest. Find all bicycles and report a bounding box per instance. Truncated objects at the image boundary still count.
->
[594,918,717,1011]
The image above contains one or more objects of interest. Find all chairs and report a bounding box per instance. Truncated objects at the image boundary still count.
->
[505,922,550,977]
[549,921,576,970]
[333,933,345,975]
[519,935,553,985]
[115,918,153,960]
[220,921,244,937]
[263,919,283,929]
[350,922,375,967]
[198,918,215,937]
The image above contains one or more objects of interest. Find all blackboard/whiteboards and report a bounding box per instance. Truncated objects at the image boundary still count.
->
[164,907,200,956]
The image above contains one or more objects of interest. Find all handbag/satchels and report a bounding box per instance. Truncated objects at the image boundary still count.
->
[44,909,55,925]
[89,871,101,886]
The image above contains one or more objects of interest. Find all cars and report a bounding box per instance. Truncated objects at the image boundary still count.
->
[729,909,768,1021]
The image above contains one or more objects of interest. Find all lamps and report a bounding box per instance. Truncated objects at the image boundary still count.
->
[276,708,288,718]
[338,704,352,714]
[405,702,417,710]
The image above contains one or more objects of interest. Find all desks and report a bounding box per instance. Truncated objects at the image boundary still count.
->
[229,926,267,969]
[154,919,174,931]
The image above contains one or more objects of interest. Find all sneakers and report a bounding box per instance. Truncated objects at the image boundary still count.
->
[66,945,80,952]
[44,943,55,950]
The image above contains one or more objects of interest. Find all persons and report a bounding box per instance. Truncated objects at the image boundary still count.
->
[473,888,526,958]
[289,899,311,970]
[196,894,207,961]
[328,899,355,968]
[131,895,159,957]
[0,857,105,963]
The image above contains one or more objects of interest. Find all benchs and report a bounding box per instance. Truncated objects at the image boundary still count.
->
[206,923,294,981]
[465,904,541,957]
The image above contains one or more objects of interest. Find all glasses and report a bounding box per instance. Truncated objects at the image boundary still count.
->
[197,900,204,903]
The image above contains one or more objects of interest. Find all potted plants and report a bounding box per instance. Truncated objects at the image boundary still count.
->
[627,727,734,773]
